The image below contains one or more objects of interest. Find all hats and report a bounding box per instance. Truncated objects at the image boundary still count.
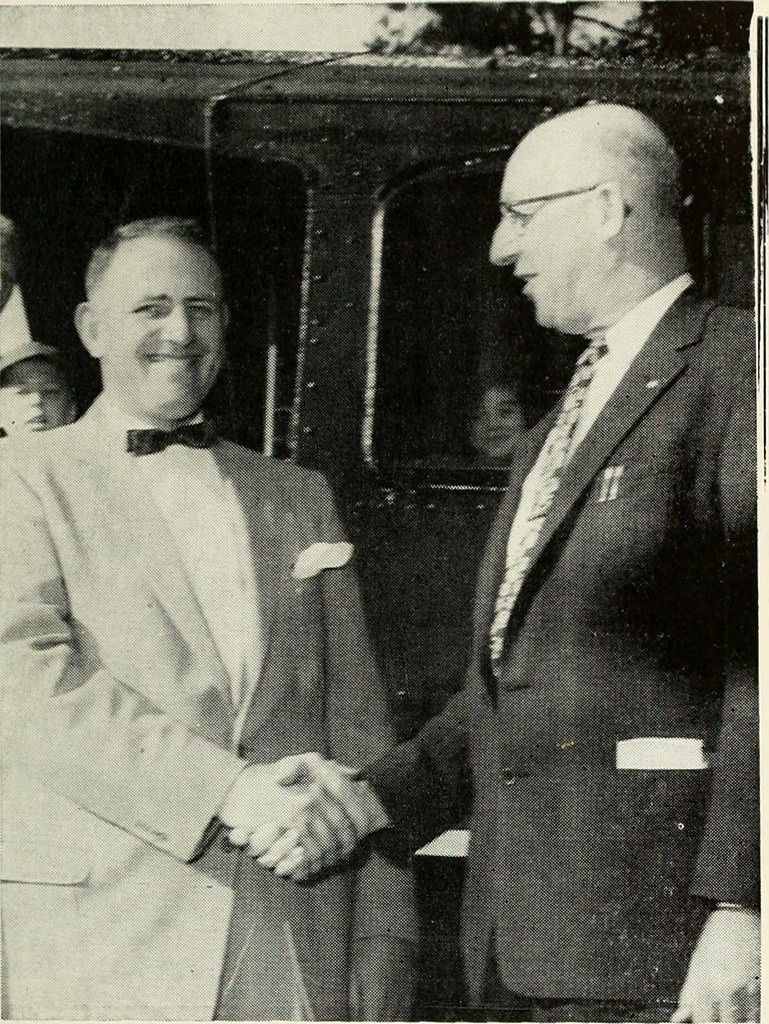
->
[0,342,68,372]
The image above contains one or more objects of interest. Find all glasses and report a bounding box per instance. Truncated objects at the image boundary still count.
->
[498,185,632,236]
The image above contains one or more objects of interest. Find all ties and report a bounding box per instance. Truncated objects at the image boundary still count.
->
[488,337,608,679]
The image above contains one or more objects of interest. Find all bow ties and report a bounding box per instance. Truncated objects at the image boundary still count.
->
[125,421,216,457]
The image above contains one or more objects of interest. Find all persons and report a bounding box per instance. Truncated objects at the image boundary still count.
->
[0,341,77,438]
[458,367,529,460]
[230,101,760,1022]
[0,219,423,1022]
